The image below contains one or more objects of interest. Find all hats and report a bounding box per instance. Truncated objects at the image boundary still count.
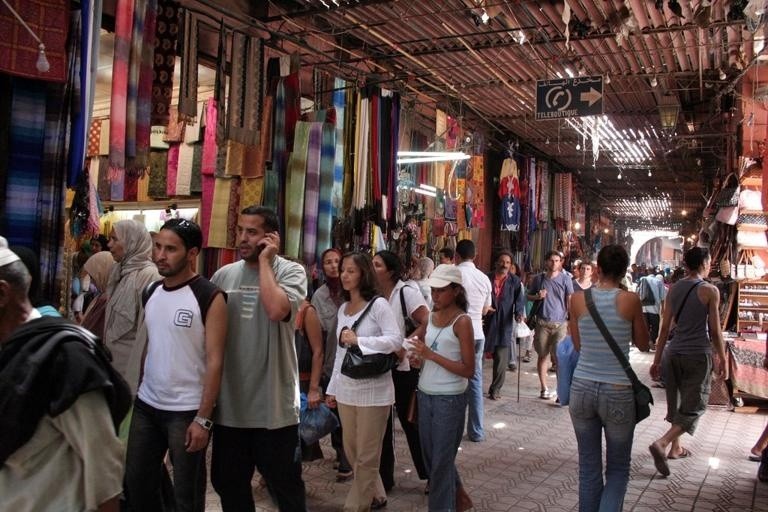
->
[423,264,463,288]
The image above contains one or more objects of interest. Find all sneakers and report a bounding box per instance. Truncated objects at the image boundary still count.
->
[523,354,533,362]
[333,459,353,478]
[508,363,517,369]
[457,488,472,511]
[371,496,387,510]
[540,391,551,398]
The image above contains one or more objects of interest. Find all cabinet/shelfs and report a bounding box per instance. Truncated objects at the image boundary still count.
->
[737,281,768,334]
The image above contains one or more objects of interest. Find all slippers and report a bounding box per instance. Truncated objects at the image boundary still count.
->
[668,446,694,459]
[649,446,670,476]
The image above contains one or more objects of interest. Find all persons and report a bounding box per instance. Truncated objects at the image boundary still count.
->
[0,203,600,510]
[619,262,689,388]
[648,245,730,476]
[748,330,768,461]
[564,242,653,510]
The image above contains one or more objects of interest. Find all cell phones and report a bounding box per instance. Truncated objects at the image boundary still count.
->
[256,233,275,251]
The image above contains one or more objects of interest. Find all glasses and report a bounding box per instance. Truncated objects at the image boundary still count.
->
[166,218,191,232]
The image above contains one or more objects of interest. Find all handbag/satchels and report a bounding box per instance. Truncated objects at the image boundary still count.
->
[698,159,768,285]
[341,343,398,378]
[295,328,328,373]
[526,318,537,330]
[408,390,419,425]
[632,380,654,423]
[405,317,416,337]
